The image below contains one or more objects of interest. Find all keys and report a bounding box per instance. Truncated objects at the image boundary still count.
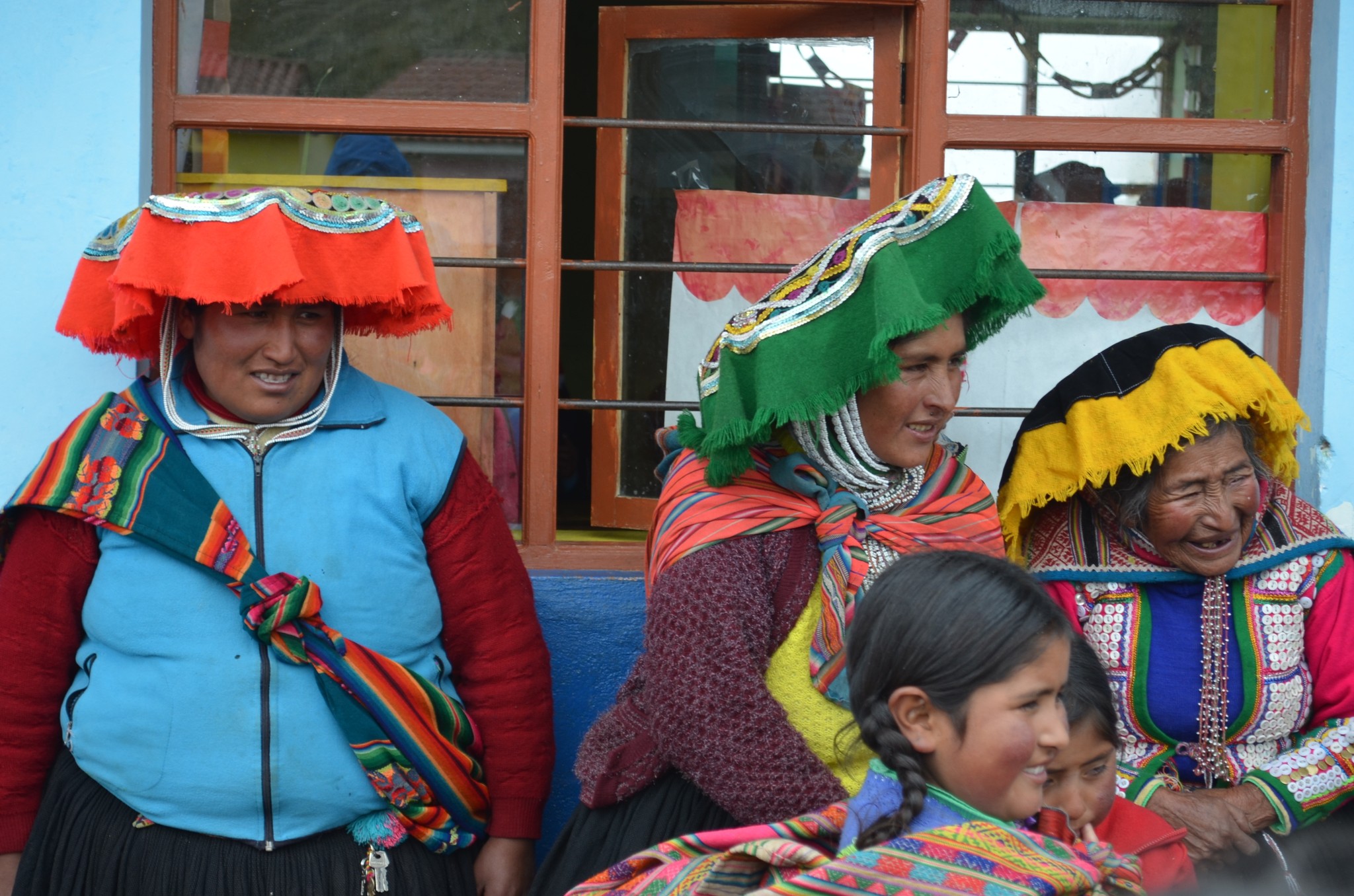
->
[361,851,390,896]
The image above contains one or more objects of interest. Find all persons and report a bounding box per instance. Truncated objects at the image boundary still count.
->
[529,174,1047,896]
[563,549,1148,896]
[0,187,556,896]
[997,322,1354,896]
[1040,633,1198,896]
